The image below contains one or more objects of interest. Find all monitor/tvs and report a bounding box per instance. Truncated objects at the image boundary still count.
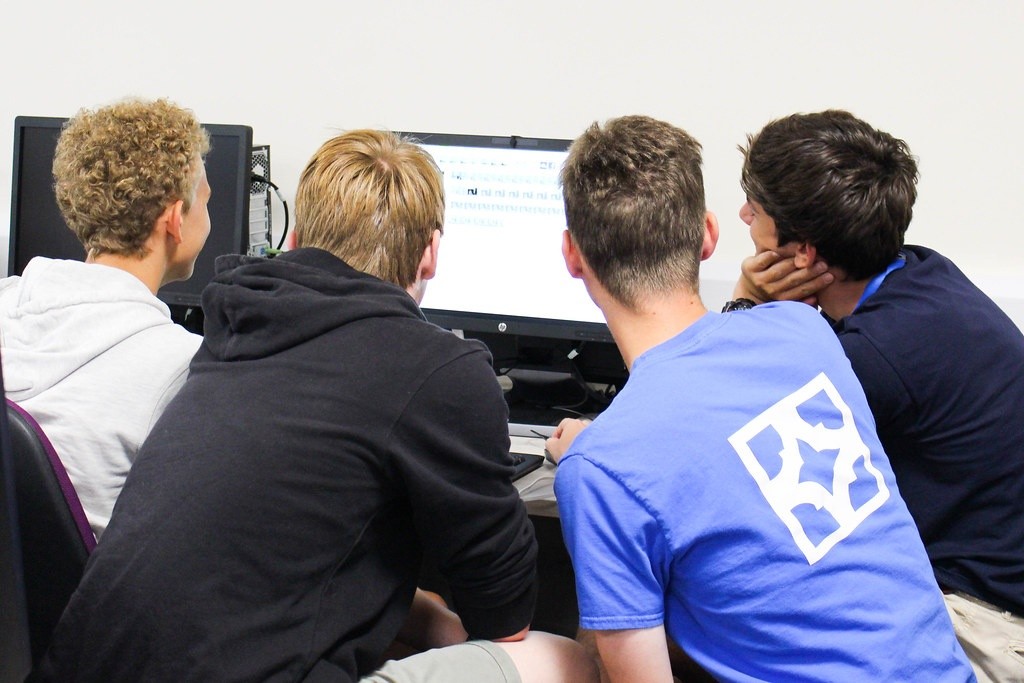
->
[389,130,631,425]
[7,114,252,307]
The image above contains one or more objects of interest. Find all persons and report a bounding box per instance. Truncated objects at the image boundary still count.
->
[22,129,599,683]
[0,99,213,544]
[545,116,976,683]
[720,110,1024,683]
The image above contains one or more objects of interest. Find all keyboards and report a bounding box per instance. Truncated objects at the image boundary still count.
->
[508,452,545,482]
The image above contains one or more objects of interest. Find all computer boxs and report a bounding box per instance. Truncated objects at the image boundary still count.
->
[169,144,271,336]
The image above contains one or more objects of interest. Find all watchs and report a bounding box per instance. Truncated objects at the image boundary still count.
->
[721,298,757,314]
